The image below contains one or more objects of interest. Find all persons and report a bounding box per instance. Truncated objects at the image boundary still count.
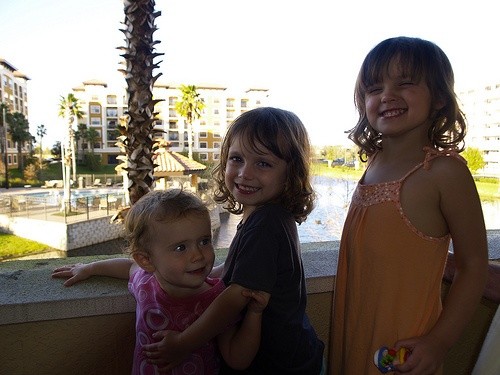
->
[138,105,328,375]
[328,35,490,375]
[50,187,272,375]
